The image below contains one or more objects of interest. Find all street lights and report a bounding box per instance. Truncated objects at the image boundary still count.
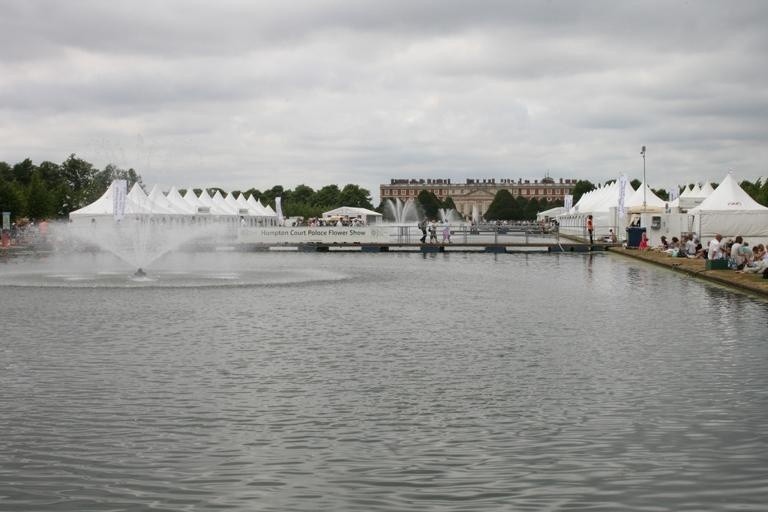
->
[640,145,646,207]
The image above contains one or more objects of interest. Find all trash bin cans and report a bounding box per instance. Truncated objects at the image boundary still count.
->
[626,227,646,249]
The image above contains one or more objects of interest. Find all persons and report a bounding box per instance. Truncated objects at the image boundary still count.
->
[428,219,440,243]
[588,215,595,243]
[608,228,617,242]
[291,214,366,226]
[12,218,52,243]
[418,217,429,242]
[640,232,649,250]
[659,234,768,278]
[240,216,245,223]
[442,218,453,243]
[467,216,559,233]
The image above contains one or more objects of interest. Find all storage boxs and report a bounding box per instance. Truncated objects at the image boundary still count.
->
[705,259,728,271]
